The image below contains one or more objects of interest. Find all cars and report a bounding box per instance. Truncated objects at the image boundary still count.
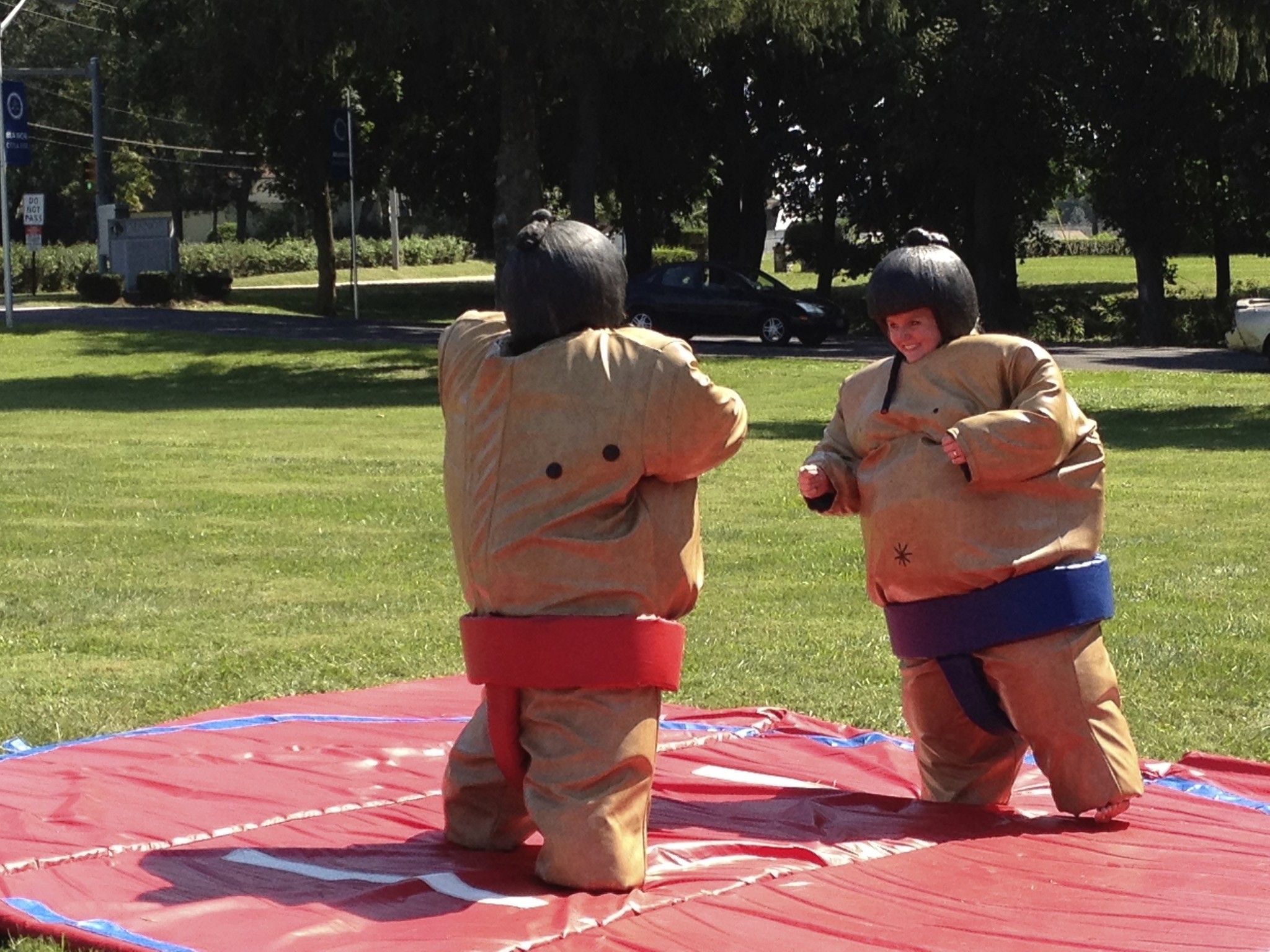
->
[621,260,826,347]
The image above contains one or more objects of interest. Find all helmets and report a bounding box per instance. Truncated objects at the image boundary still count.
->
[866,228,980,344]
[494,209,628,353]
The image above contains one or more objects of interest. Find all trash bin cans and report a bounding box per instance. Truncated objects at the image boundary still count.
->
[104,217,179,292]
[772,242,787,272]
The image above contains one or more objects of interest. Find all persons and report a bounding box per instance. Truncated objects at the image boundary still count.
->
[796,226,1148,824]
[437,209,747,889]
